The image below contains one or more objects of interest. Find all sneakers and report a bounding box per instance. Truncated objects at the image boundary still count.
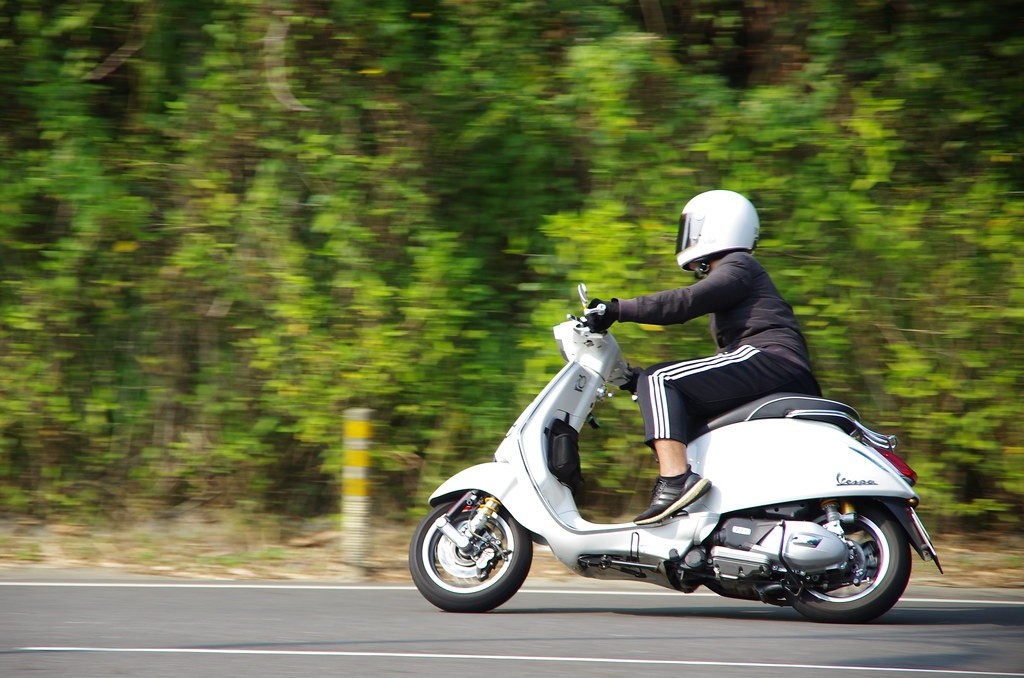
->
[633,464,712,525]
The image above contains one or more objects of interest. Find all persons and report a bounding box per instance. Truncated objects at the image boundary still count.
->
[585,190,824,525]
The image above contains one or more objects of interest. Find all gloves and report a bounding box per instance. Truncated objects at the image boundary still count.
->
[585,297,618,332]
[619,366,644,392]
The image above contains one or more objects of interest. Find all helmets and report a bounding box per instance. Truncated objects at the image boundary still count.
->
[675,190,760,271]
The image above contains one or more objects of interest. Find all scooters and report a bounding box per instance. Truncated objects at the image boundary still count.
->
[407,282,945,625]
